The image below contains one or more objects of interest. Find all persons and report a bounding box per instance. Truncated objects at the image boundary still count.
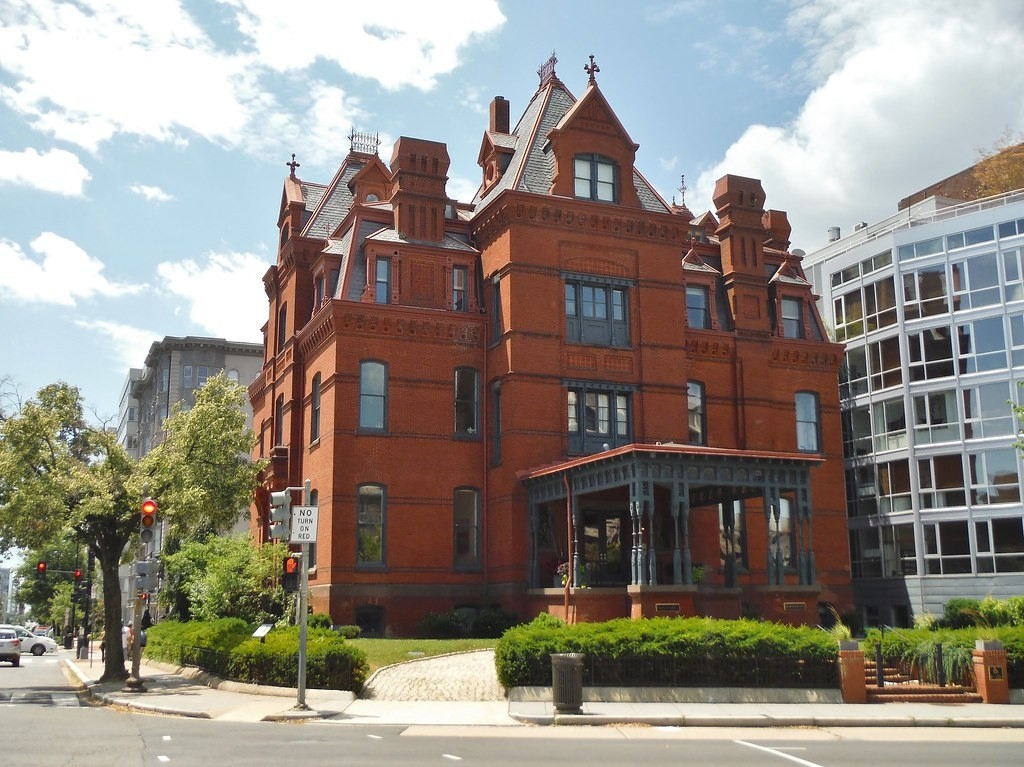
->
[141,626,147,655]
[101,625,106,666]
[121,619,134,661]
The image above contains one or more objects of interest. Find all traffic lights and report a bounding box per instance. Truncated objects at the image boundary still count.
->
[70,593,78,603]
[74,569,80,588]
[267,491,290,541]
[79,580,87,595]
[139,499,157,543]
[284,557,299,576]
[37,562,45,580]
[135,557,158,590]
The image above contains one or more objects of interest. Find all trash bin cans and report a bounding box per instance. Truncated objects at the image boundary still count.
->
[64,634,73,648]
[77,637,89,659]
[550,653,585,714]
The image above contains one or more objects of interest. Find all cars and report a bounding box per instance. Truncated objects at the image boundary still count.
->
[0,629,21,666]
[0,625,57,656]
